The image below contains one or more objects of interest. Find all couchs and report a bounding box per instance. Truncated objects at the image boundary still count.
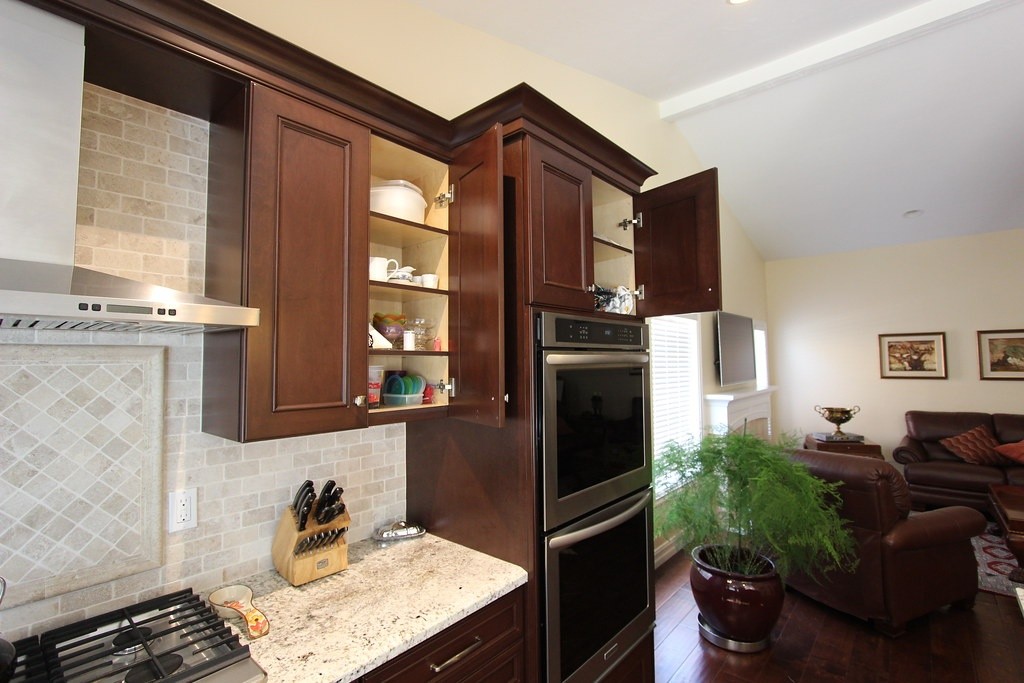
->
[892,411,1024,510]
[769,448,985,637]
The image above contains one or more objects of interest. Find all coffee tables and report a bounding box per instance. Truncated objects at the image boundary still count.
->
[986,483,1024,582]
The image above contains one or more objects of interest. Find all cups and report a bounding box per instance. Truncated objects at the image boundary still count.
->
[414,274,438,288]
[369,257,399,282]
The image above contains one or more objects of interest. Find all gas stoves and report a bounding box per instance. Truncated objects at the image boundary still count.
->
[0,586,269,682]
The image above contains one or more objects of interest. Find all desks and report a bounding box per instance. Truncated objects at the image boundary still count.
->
[802,432,884,461]
[706,385,776,534]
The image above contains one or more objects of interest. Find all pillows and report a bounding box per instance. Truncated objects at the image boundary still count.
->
[940,425,1016,467]
[991,440,1024,464]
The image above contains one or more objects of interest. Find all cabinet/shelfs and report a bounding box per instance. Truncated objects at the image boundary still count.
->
[351,587,531,682]
[200,77,724,442]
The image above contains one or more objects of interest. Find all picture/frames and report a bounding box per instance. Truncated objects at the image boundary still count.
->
[977,330,1024,380]
[877,333,947,379]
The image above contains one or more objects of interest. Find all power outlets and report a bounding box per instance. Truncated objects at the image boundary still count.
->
[169,488,198,533]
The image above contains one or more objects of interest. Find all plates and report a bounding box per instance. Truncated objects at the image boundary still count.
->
[385,375,426,404]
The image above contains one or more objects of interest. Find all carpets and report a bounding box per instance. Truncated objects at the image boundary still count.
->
[907,507,1024,595]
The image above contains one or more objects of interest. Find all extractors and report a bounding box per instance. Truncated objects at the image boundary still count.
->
[0,0,262,343]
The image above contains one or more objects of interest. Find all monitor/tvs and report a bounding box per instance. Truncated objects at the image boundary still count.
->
[715,310,757,388]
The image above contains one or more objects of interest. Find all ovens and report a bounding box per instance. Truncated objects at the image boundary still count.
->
[528,307,658,682]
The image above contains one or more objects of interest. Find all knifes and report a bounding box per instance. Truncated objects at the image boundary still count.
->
[290,479,349,555]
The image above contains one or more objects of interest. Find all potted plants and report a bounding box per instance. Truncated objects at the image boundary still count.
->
[650,428,861,653]
